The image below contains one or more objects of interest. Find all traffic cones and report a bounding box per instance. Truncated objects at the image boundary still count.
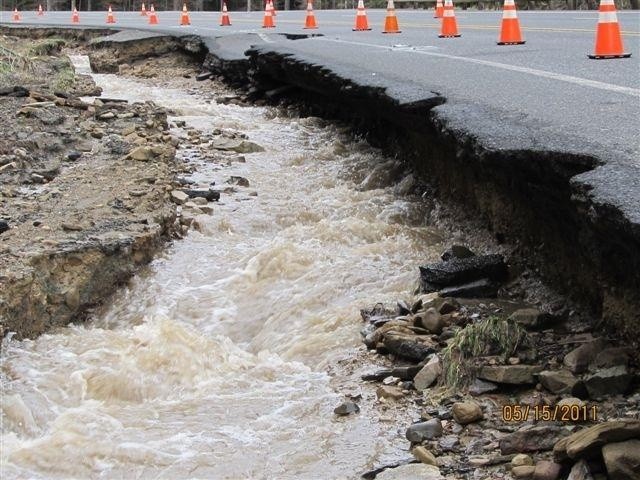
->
[351,0,463,41]
[37,4,45,17]
[104,4,117,26]
[588,0,631,60]
[70,7,81,25]
[137,0,323,31]
[498,0,527,46]
[13,7,20,23]
